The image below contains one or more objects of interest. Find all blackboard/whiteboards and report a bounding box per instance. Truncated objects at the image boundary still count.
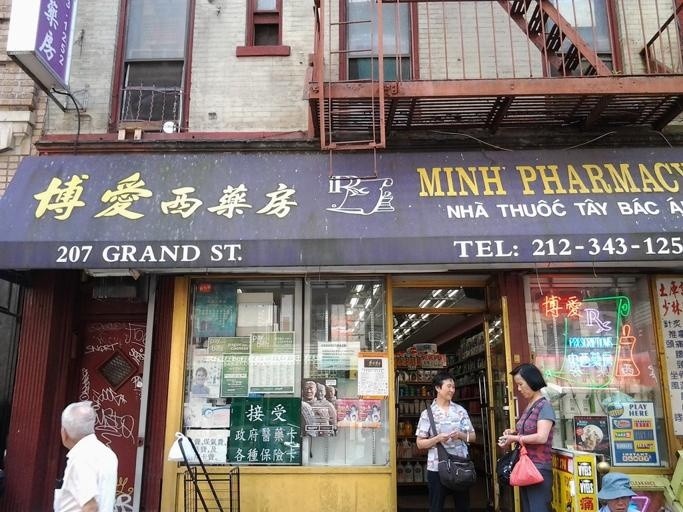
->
[228,398,303,467]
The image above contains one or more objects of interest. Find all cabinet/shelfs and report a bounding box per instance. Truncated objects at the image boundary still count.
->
[394,338,508,486]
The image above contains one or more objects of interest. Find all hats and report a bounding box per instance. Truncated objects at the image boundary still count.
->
[595,473,637,500]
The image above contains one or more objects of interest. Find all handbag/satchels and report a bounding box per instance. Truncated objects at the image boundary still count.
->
[495,449,519,489]
[437,444,477,491]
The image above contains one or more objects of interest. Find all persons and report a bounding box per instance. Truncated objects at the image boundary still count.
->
[595,472,637,512]
[324,384,336,410]
[190,366,209,394]
[413,372,476,512]
[302,380,329,437]
[314,382,336,435]
[52,402,119,512]
[496,363,557,511]
